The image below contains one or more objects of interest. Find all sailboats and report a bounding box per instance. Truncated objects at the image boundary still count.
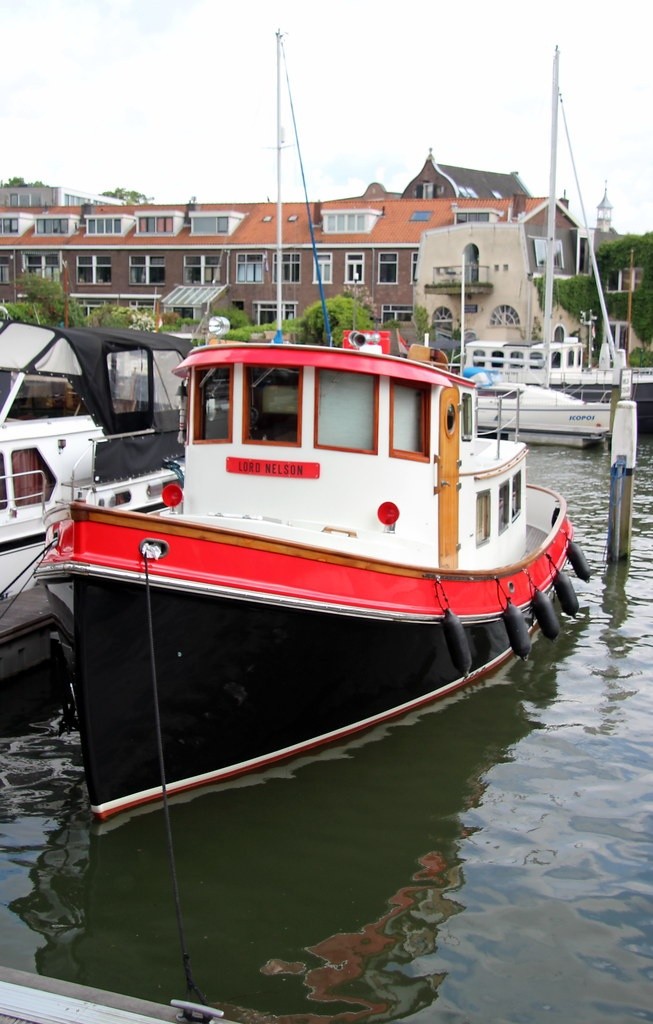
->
[457,44,653,419]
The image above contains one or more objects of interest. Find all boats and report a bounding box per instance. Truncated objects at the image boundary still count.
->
[0,28,576,822]
[463,379,616,451]
